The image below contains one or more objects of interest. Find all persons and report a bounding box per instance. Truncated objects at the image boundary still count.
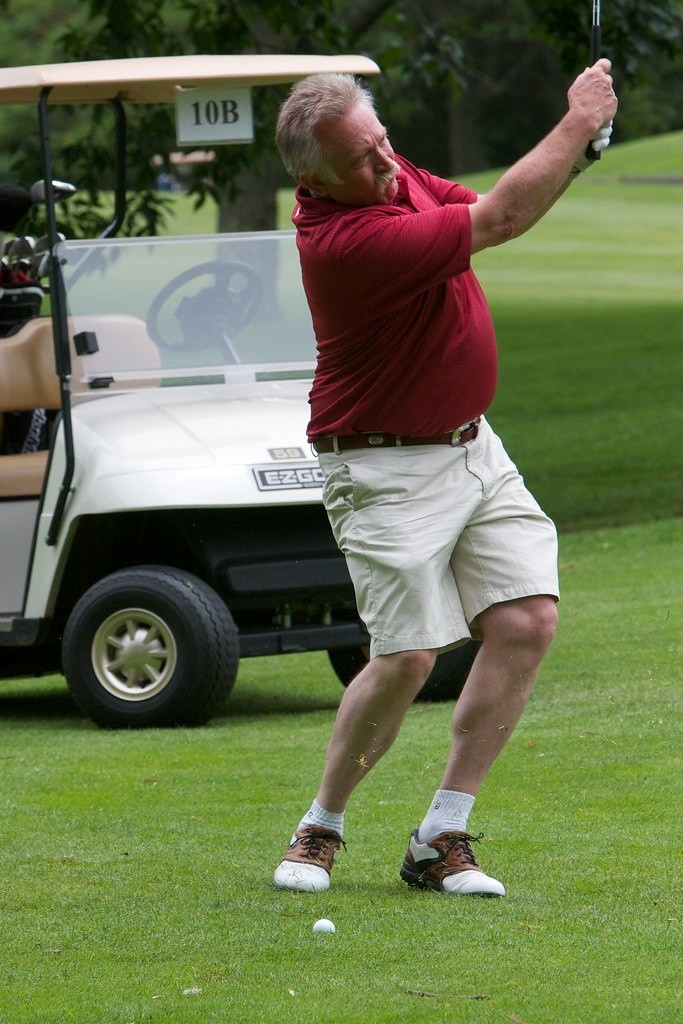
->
[275,58,618,895]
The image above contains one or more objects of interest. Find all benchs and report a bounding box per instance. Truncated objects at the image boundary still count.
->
[0,314,159,500]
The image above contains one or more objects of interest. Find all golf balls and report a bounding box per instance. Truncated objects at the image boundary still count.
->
[313,918,335,934]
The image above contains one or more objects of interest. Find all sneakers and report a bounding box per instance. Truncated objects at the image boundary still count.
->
[273,825,347,894]
[399,829,505,898]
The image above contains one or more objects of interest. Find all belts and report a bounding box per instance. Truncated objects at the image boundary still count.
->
[316,416,481,453]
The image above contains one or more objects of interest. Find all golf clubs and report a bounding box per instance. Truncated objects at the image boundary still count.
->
[0,179,77,284]
[585,0,601,161]
